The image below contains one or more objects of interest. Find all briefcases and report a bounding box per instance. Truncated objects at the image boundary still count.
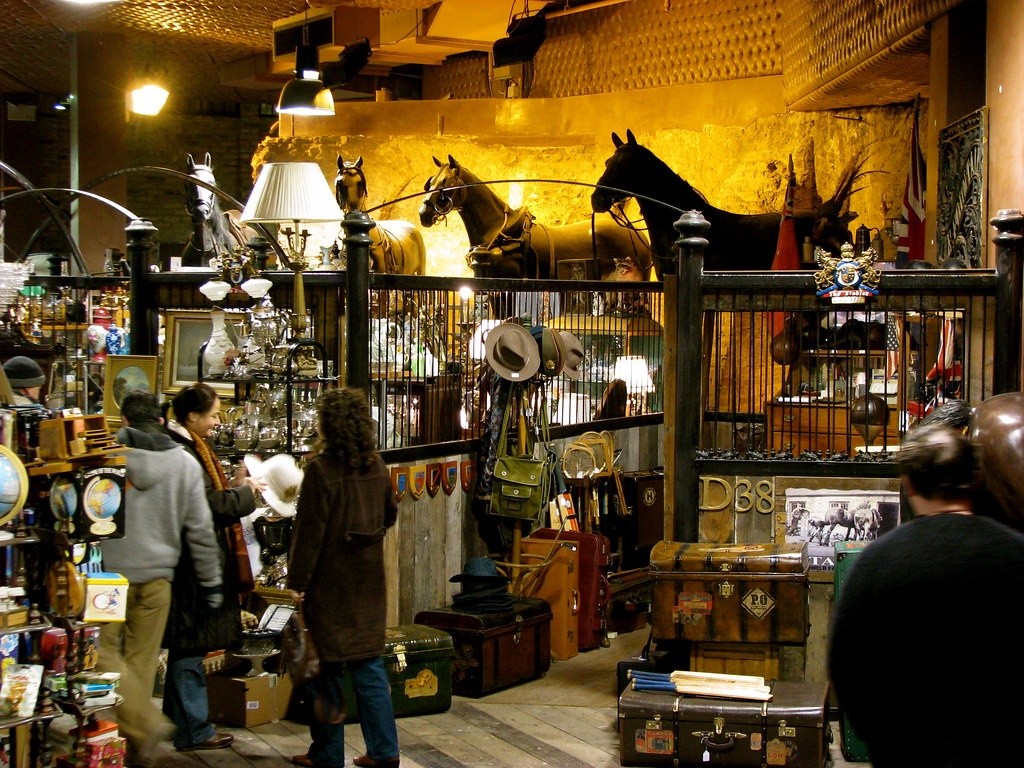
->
[518,538,580,660]
[530,527,611,652]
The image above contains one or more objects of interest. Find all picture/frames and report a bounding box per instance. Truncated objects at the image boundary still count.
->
[161,309,251,399]
[103,354,160,427]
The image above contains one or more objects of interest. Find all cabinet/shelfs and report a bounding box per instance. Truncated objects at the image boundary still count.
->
[0,614,62,768]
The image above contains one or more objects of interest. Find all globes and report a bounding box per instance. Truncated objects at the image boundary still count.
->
[82,476,121,535]
[50,475,78,534]
[0,444,29,541]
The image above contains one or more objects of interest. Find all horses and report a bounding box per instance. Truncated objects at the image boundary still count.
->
[591,128,852,282]
[419,153,650,319]
[182,152,260,265]
[336,155,426,317]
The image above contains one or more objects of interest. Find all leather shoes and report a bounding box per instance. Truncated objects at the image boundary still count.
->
[293,754,313,767]
[177,732,236,750]
[353,755,400,768]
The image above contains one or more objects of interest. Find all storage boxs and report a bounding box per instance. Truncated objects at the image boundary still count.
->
[85,736,128,760]
[343,623,455,724]
[224,671,293,728]
[56,755,90,768]
[414,598,553,699]
[83,756,125,768]
[82,572,129,622]
[40,414,133,461]
[834,539,868,598]
[67,719,120,752]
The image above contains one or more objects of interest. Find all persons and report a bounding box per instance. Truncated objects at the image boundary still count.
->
[3,356,45,404]
[827,424,1024,768]
[293,389,400,768]
[159,383,268,752]
[100,391,223,764]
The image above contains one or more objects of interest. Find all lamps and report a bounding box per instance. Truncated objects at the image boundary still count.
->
[238,162,345,378]
[273,0,336,115]
[609,354,656,416]
[851,393,891,446]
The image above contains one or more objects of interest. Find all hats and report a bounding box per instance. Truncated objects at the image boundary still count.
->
[450,558,514,612]
[244,453,304,517]
[3,356,45,388]
[485,322,583,381]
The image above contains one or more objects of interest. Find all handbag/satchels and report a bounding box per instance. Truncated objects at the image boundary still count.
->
[279,597,320,680]
[489,446,556,520]
